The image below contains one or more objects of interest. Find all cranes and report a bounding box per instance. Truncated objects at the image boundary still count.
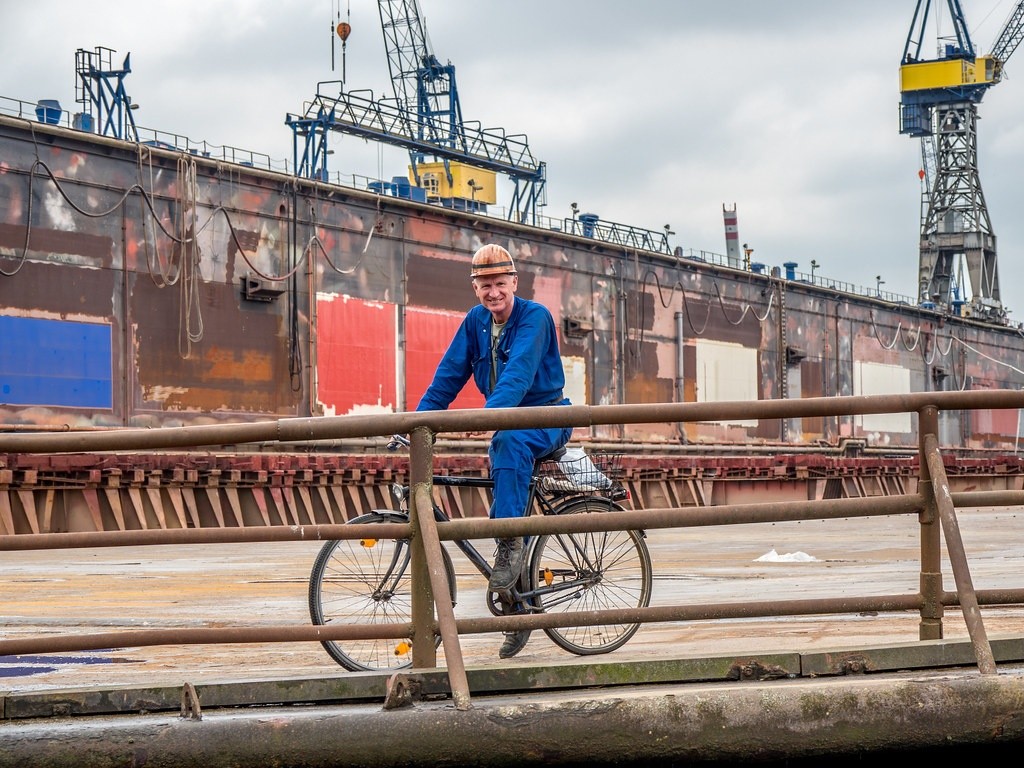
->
[324,0,498,214]
[888,0,1024,328]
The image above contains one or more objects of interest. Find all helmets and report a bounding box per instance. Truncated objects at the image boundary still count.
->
[470,244,517,276]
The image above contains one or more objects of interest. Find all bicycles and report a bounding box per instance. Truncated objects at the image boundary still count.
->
[304,434,653,674]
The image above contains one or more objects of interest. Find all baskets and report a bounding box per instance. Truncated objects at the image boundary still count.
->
[542,451,627,492]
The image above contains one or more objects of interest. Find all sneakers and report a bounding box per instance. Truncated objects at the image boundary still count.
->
[489,534,528,592]
[499,627,533,659]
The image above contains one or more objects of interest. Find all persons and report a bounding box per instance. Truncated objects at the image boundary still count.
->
[417,244,573,659]
